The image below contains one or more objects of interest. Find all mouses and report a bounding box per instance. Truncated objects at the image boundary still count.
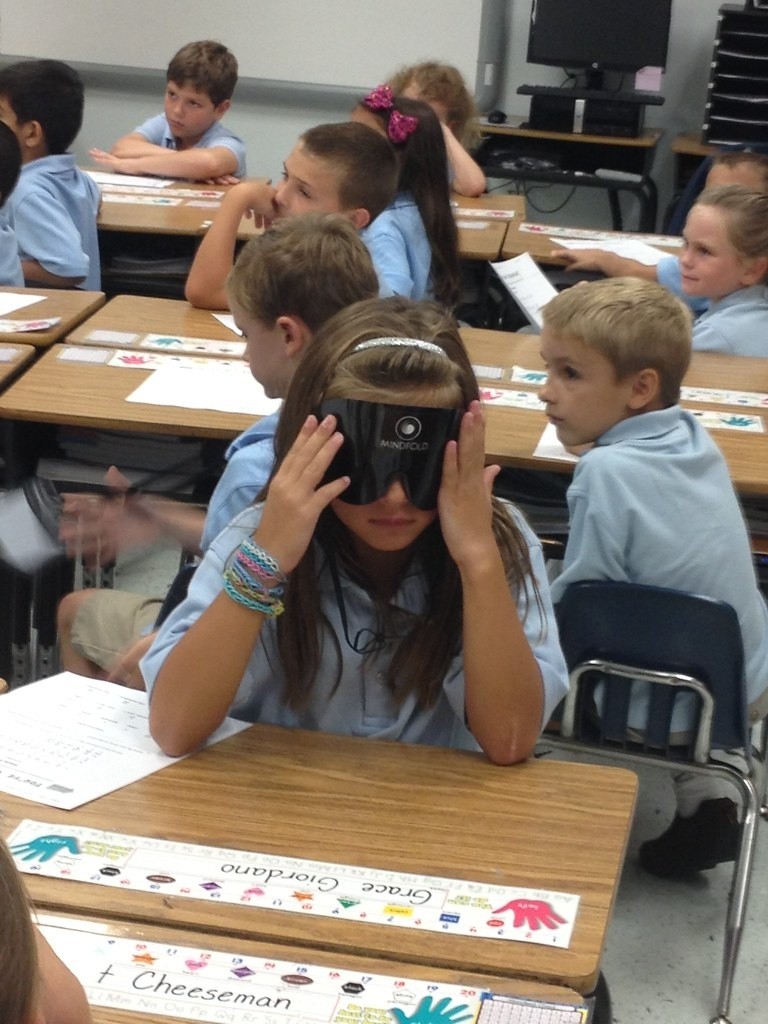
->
[487,109,507,124]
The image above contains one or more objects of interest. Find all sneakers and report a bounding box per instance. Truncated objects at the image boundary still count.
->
[640,796,747,877]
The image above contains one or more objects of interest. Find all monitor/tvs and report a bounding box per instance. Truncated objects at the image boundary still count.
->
[526,0,673,89]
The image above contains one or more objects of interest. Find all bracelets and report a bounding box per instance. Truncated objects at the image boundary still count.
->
[223,538,286,619]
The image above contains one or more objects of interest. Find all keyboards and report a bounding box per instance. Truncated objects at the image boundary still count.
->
[517,84,665,106]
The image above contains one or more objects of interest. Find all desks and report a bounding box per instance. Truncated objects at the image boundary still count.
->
[0,117,768,1024]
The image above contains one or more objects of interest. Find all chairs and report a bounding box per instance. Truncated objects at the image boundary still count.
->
[534,580,759,1023]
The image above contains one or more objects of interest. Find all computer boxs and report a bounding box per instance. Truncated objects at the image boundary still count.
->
[528,95,646,137]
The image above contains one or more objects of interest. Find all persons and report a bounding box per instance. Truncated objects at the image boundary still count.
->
[55,212,375,690]
[90,40,247,186]
[386,63,486,199]
[140,295,569,763]
[351,86,462,306]
[538,276,767,876]
[1,838,93,1024]
[0,61,103,291]
[553,150,768,360]
[186,122,398,310]
